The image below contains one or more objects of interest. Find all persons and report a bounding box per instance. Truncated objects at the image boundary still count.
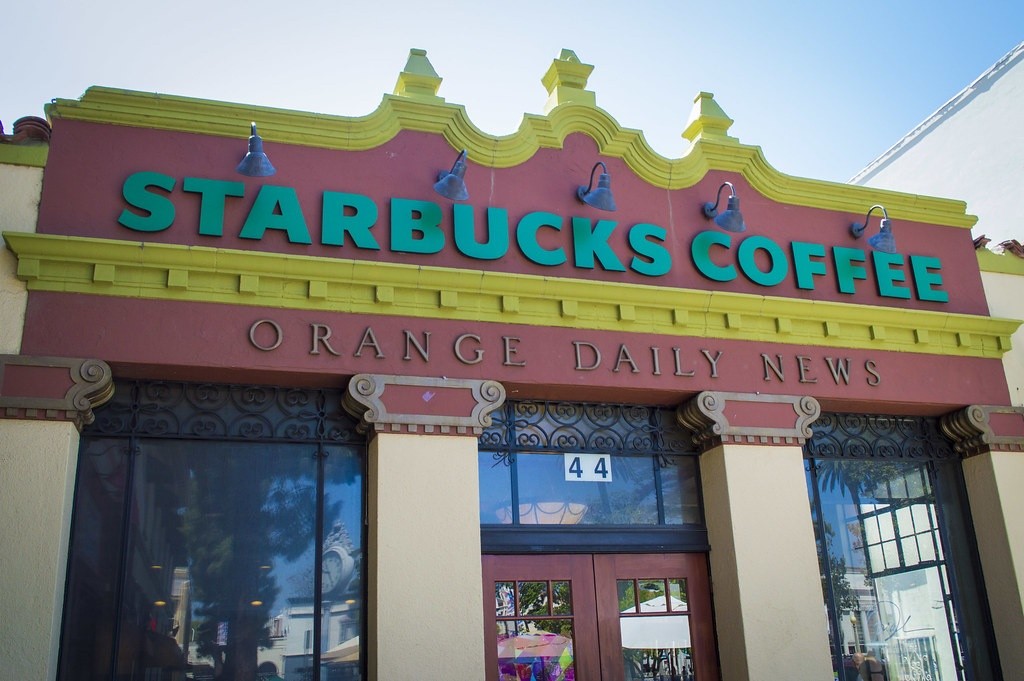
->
[256,662,285,681]
[853,651,889,681]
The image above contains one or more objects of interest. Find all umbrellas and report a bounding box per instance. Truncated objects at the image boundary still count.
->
[620,594,692,650]
[308,633,360,667]
[498,629,575,681]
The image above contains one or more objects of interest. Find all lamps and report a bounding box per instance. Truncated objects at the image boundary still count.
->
[704,180,747,234]
[432,149,471,200]
[850,204,898,255]
[576,160,615,212]
[234,122,278,177]
